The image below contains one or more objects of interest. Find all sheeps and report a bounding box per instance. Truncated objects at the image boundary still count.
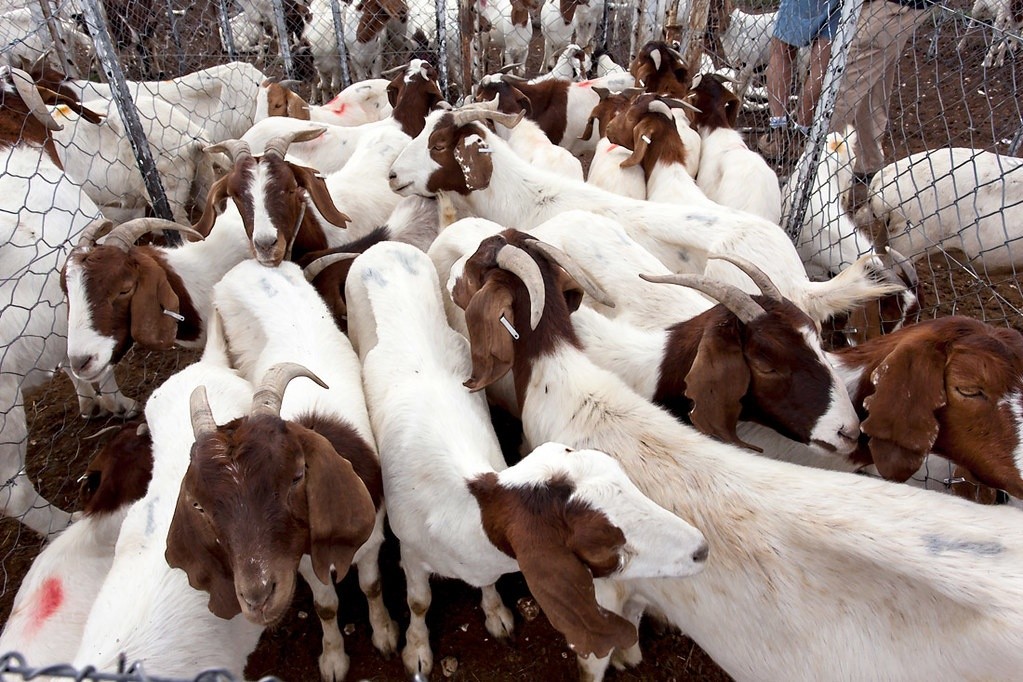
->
[1,0,1020,682]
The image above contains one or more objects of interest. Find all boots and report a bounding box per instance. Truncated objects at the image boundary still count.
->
[787,129,807,162]
[758,127,786,159]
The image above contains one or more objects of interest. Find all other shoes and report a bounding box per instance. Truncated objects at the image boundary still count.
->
[847,183,869,202]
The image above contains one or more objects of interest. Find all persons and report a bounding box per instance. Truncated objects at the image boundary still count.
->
[755,1,842,164]
[828,1,944,183]
